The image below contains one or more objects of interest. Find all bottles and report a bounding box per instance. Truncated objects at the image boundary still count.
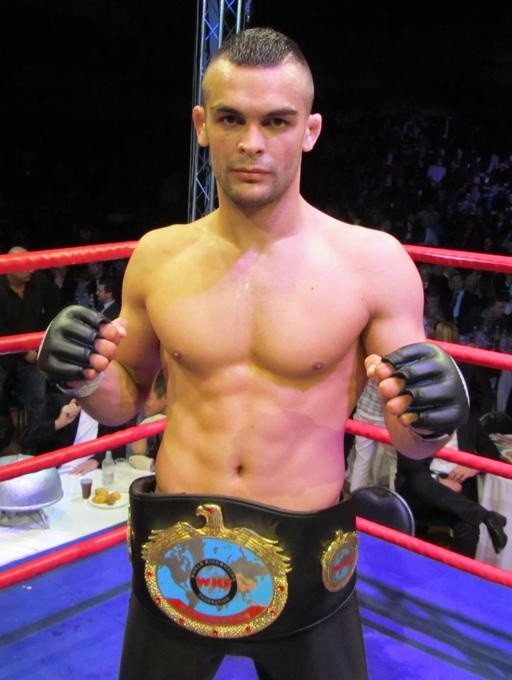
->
[102,450,115,489]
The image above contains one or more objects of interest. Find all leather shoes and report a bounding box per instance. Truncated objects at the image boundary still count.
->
[486,510,508,553]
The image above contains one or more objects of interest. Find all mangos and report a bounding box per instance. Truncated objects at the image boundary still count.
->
[92,488,121,504]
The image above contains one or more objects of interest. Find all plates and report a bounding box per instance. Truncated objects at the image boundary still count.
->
[88,492,129,508]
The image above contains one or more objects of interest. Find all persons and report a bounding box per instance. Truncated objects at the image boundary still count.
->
[0,223,167,476]
[38,48,469,680]
[326,138,511,414]
[396,413,508,562]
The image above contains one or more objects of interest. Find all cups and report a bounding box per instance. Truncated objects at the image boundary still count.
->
[80,478,93,499]
[115,458,126,472]
